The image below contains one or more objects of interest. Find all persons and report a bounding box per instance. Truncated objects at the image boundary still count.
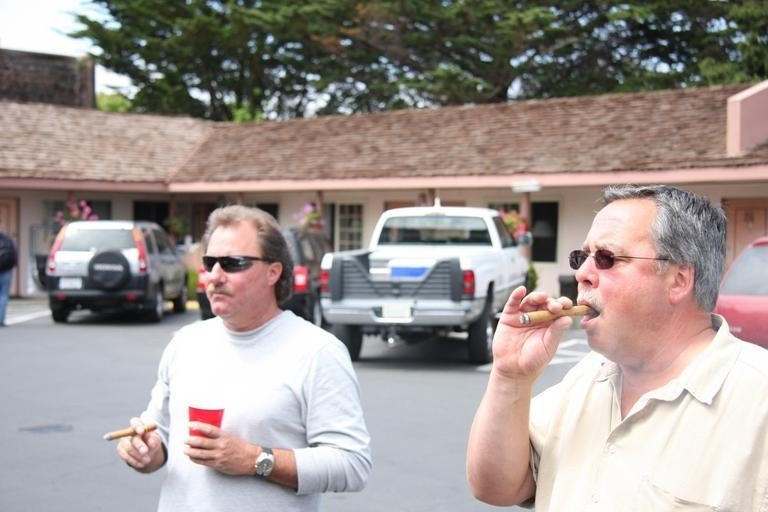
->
[0,230,17,329]
[461,182,765,512]
[112,201,375,512]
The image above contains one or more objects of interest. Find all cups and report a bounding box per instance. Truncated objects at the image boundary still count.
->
[189,405,224,452]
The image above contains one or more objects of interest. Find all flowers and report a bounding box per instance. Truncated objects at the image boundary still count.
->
[49,191,100,234]
[500,205,529,241]
[290,202,325,232]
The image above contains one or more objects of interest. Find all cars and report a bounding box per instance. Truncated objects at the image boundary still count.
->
[708,233,768,351]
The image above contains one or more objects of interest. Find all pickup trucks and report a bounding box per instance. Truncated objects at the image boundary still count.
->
[316,195,535,368]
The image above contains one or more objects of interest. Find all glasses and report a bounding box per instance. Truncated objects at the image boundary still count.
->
[567,248,674,270]
[201,255,267,272]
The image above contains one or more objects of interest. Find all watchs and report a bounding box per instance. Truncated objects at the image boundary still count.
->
[254,443,274,481]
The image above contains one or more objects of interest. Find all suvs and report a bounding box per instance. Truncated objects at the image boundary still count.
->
[26,218,192,325]
[193,227,340,330]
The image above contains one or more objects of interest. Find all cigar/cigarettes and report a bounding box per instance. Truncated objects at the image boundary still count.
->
[103,423,157,442]
[519,304,595,325]
